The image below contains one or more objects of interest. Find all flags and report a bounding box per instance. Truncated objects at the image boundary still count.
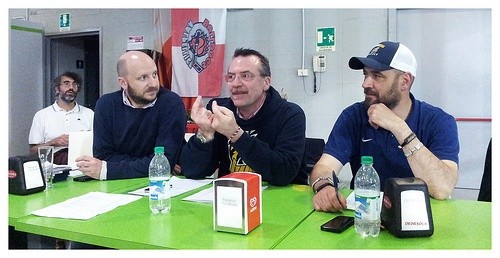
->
[170,9,228,97]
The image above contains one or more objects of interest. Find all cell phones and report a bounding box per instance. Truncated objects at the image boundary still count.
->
[322,215,354,234]
[73,175,93,182]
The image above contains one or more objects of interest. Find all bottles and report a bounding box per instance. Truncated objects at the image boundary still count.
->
[149,147,172,215]
[354,156,380,238]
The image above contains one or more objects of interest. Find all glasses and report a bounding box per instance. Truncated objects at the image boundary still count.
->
[224,71,271,83]
[59,81,80,88]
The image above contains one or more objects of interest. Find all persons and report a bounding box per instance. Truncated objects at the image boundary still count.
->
[75,51,186,181]
[29,71,95,178]
[312,41,461,213]
[181,46,309,187]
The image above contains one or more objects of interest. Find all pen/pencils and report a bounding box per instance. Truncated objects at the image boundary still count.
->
[332,170,340,202]
[144,184,173,192]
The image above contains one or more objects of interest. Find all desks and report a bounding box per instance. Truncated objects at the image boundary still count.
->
[9,175,491,250]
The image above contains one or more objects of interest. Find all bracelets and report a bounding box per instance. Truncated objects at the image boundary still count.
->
[314,183,335,193]
[398,133,417,149]
[227,127,241,140]
[404,142,423,157]
[312,175,334,187]
[196,132,207,143]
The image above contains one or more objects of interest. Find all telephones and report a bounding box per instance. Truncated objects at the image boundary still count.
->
[312,55,326,72]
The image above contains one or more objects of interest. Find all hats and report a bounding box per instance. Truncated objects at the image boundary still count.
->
[349,41,417,78]
